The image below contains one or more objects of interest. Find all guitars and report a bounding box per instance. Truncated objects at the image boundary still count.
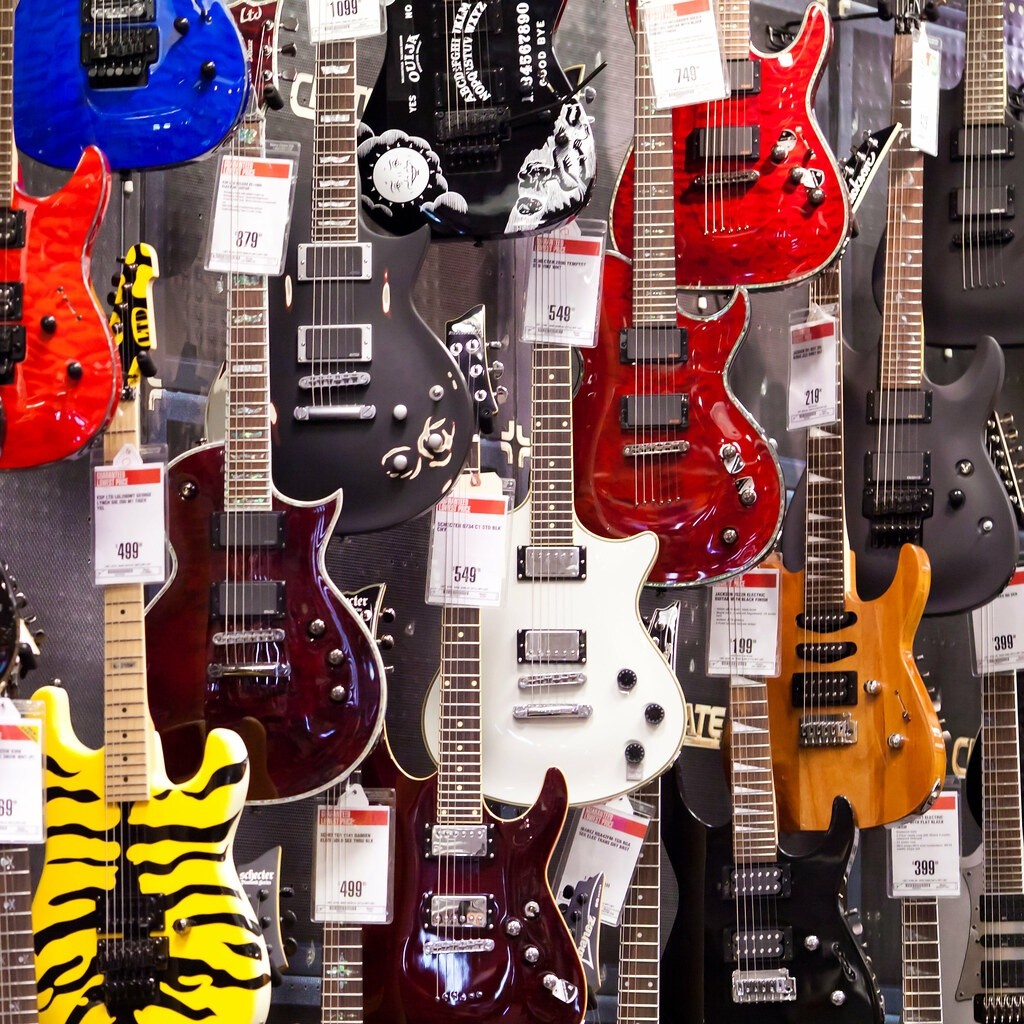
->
[14,1,253,176]
[30,243,274,1023]
[615,598,682,1023]
[873,1,1024,348]
[970,671,1023,1023]
[778,0,1019,619]
[236,843,298,1024]
[0,554,46,1024]
[139,2,389,812]
[608,0,853,294]
[271,0,473,531]
[364,299,590,1024]
[571,11,786,589]
[659,547,887,1024]
[900,896,944,1022]
[30,236,273,1023]
[320,758,365,1023]
[417,216,692,812]
[353,2,599,241]
[0,1,121,470]
[720,123,950,835]
